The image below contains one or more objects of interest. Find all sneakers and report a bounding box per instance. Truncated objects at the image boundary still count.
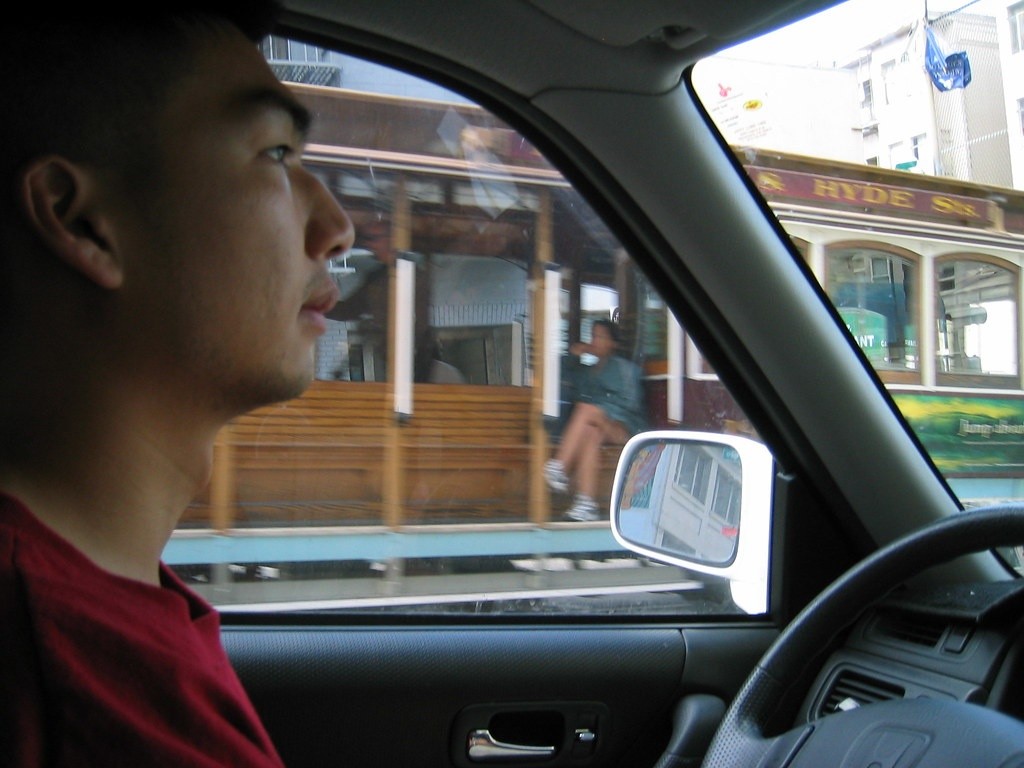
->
[562,491,601,521]
[545,458,571,495]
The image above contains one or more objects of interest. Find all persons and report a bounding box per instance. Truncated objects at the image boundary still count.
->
[543,319,650,522]
[0,0,356,768]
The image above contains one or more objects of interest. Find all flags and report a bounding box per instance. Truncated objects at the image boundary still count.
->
[924,28,971,91]
